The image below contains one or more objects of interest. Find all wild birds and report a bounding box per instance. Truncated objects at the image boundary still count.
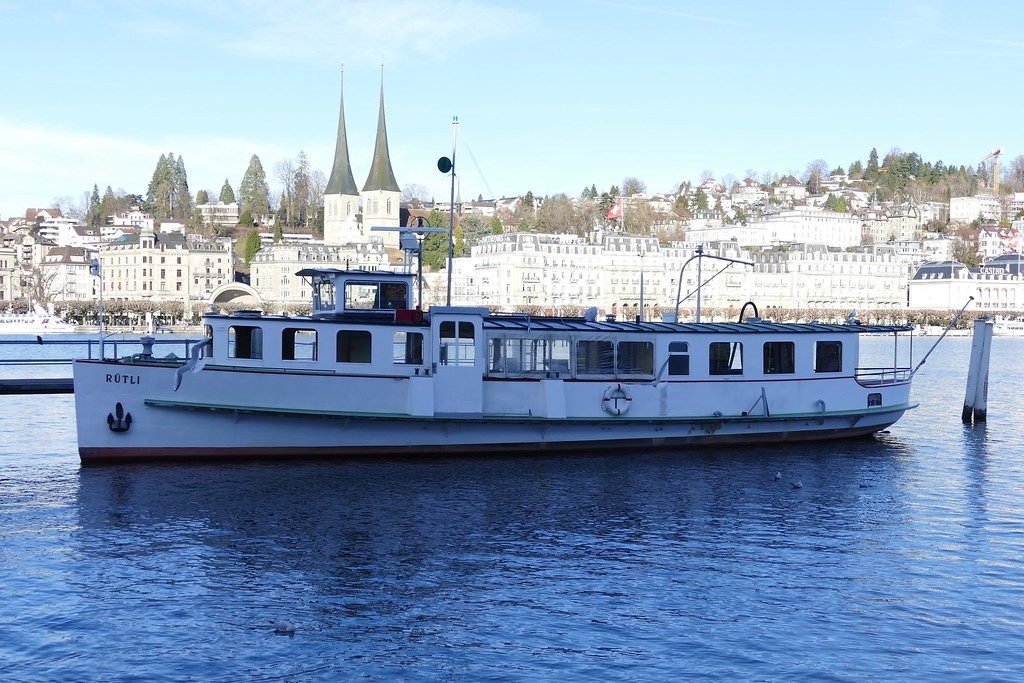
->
[774,471,782,481]
[789,479,803,489]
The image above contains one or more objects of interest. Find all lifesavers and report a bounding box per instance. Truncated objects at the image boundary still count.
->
[603,383,633,416]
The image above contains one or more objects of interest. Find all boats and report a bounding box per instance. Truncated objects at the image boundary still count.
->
[72,113,996,463]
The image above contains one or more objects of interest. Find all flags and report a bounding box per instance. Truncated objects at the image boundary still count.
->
[607,201,622,218]
